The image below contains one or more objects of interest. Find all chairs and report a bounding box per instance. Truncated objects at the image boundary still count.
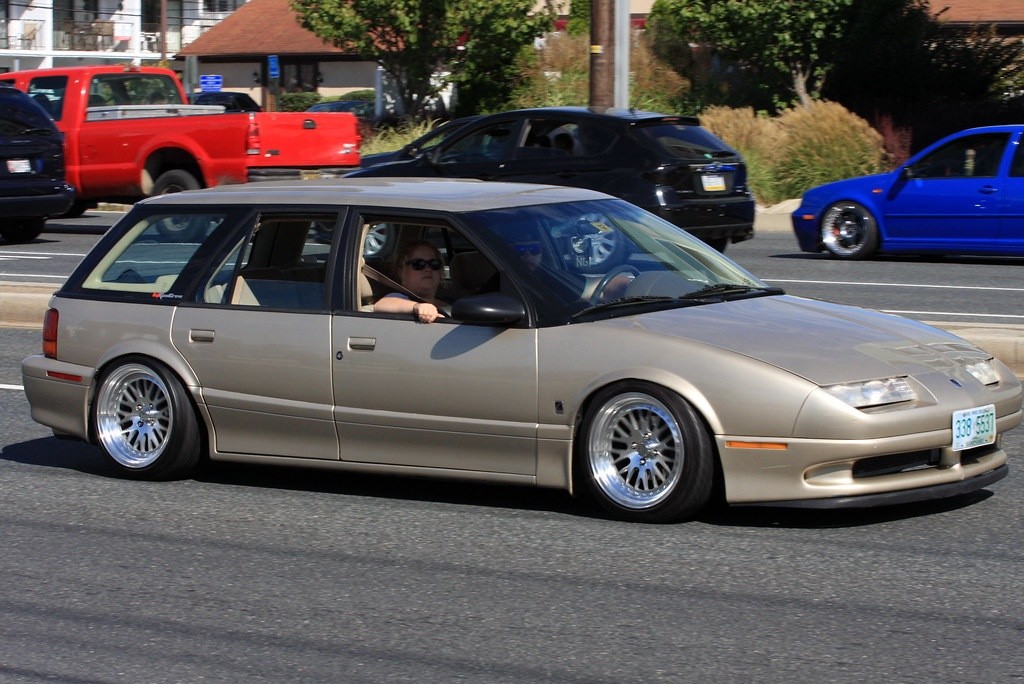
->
[71,19,115,50]
[449,251,499,298]
[536,132,574,156]
[362,261,397,308]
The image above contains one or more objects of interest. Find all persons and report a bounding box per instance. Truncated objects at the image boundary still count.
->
[490,228,631,308]
[374,239,451,324]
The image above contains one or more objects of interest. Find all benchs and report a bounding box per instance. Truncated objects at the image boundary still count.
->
[205,265,323,306]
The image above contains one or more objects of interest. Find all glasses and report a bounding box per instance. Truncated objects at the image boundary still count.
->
[403,257,443,271]
[510,243,543,256]
